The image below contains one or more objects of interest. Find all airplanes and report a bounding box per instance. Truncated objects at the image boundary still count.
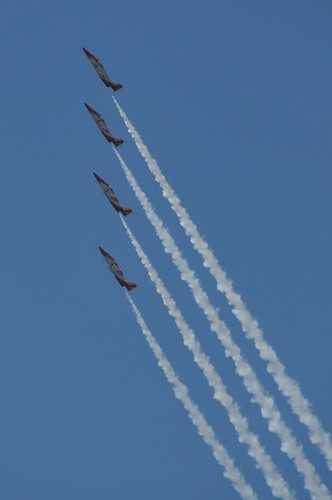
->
[83,45,124,93]
[94,172,133,218]
[83,101,125,148]
[96,245,137,293]
